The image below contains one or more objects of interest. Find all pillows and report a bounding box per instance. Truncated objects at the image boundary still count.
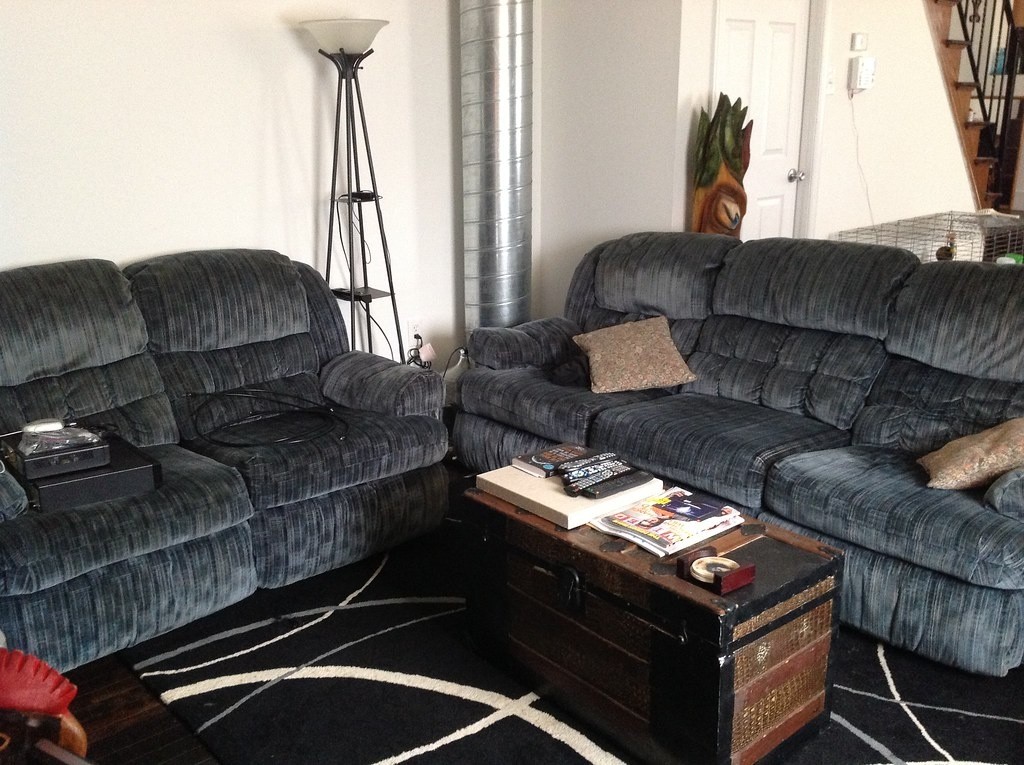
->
[572,314,698,394]
[916,416,1023,490]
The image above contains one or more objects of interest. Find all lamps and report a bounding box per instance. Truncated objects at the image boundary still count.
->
[301,17,405,362]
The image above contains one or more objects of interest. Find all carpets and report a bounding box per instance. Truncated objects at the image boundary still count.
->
[112,468,1022,764]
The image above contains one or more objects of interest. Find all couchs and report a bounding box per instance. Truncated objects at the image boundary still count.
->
[452,231,1024,678]
[0,247,450,679]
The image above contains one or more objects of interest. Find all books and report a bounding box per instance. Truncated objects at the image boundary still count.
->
[511,441,604,479]
[587,485,747,558]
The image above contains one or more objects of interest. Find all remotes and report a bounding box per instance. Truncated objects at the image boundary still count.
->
[557,451,654,500]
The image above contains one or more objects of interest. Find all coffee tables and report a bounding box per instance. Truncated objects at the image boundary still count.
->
[460,481,845,765]
